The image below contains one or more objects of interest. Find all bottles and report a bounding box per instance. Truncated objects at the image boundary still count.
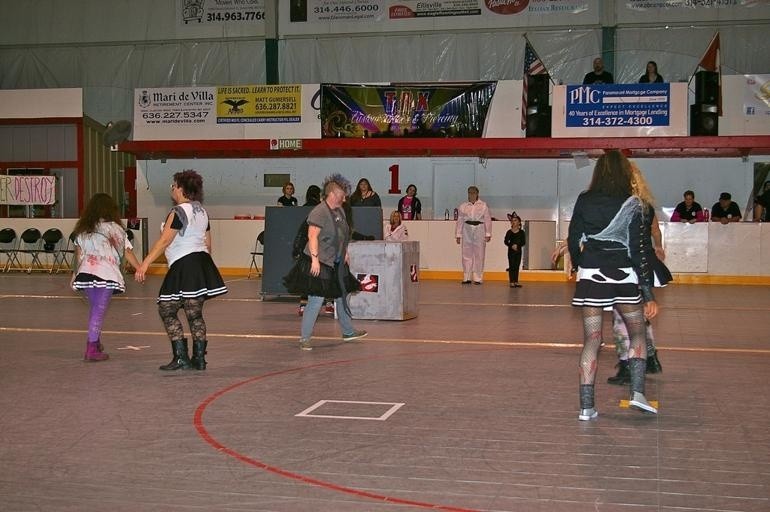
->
[445,209,449,220]
[704,209,709,222]
[454,208,458,220]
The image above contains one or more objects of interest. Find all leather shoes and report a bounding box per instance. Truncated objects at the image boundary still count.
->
[510,284,516,288]
[517,282,522,288]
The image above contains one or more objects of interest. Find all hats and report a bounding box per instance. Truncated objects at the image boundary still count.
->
[718,191,731,202]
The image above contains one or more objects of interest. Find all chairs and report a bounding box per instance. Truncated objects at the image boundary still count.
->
[30,228,71,276]
[123,229,135,274]
[248,232,264,279]
[56,231,77,275]
[7,228,45,274]
[0,228,27,274]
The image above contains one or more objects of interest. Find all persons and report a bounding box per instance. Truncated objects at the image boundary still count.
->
[639,61,664,83]
[550,161,673,386]
[302,185,322,208]
[283,175,368,351]
[582,57,614,84]
[276,182,298,206]
[297,203,375,316]
[454,186,492,285]
[70,193,139,363]
[134,170,228,372]
[752,180,770,223]
[711,192,743,224]
[670,191,704,224]
[350,178,382,206]
[398,184,422,220]
[503,211,526,289]
[567,150,659,421]
[384,210,409,242]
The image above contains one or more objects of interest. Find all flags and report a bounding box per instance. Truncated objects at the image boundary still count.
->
[521,33,551,131]
[696,30,722,116]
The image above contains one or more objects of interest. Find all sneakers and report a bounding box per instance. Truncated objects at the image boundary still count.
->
[343,329,368,342]
[628,390,658,416]
[577,408,598,421]
[298,340,313,351]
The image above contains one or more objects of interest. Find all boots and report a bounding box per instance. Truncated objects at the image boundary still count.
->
[159,338,193,371]
[85,340,110,361]
[189,340,209,371]
[606,360,630,385]
[646,349,663,375]
